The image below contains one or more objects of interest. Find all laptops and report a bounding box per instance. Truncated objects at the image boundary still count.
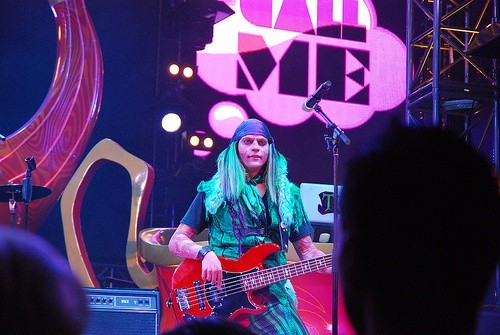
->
[299,182,342,224]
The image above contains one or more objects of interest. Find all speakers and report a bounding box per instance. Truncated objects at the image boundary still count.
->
[82,287,162,335]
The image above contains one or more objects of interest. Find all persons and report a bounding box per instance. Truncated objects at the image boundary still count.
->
[332,120,500,334]
[168,117,332,335]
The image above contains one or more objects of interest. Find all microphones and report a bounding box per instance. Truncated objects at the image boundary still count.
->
[302,81,331,112]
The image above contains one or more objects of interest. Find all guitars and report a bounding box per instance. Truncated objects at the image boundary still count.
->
[157,241,331,331]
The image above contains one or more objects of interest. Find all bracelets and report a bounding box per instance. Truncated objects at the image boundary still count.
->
[197,245,216,261]
